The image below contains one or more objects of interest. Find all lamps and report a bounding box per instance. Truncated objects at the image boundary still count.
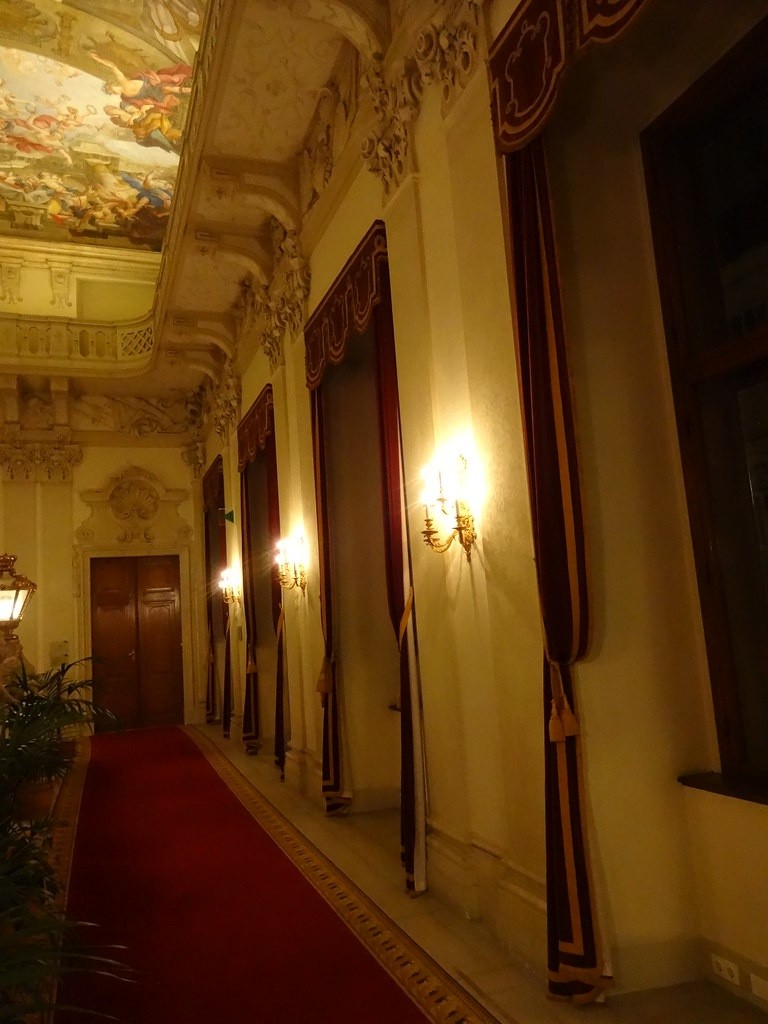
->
[0,552,37,641]
[220,569,241,609]
[420,452,477,563]
[275,535,308,597]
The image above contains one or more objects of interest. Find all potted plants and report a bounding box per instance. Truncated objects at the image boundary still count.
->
[0,815,53,950]
[0,654,116,822]
